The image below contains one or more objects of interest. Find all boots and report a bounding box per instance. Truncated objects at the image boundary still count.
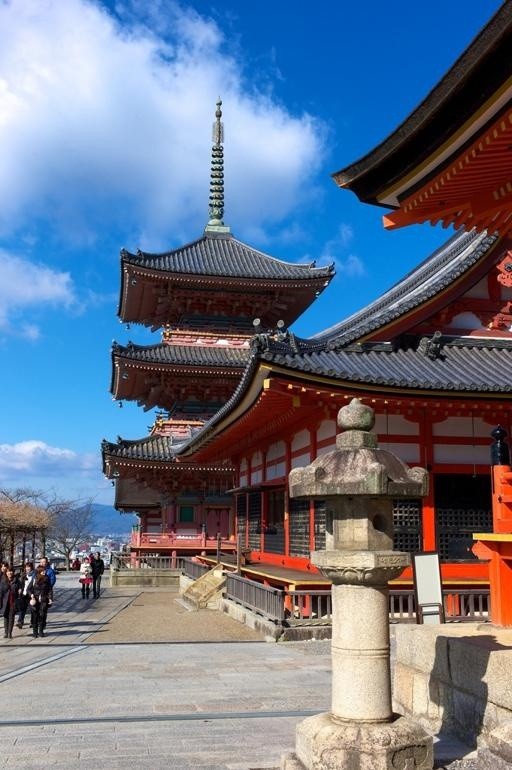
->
[81,588,90,599]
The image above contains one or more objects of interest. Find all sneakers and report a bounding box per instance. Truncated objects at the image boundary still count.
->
[3,632,12,638]
[92,594,100,599]
[33,632,48,638]
[16,622,23,629]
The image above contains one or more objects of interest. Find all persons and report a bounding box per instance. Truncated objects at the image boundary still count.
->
[92,552,104,600]
[79,557,93,599]
[0,555,58,638]
[71,558,81,571]
[89,553,94,563]
